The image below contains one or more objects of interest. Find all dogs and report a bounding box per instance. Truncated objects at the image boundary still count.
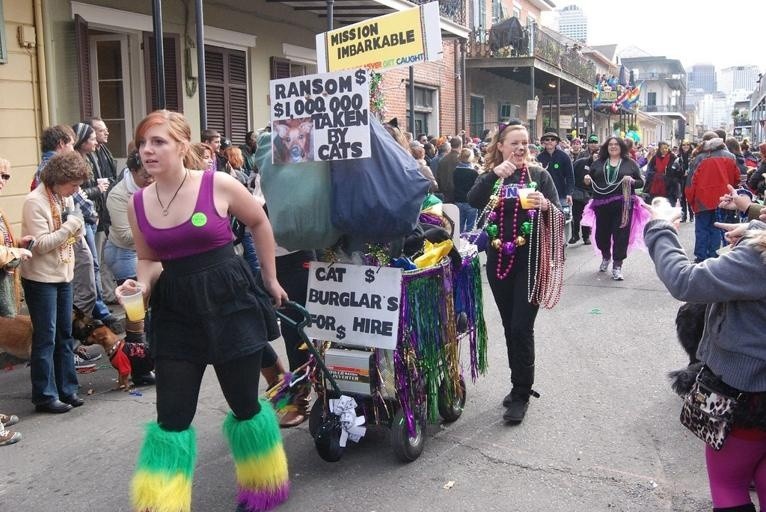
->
[82,325,154,390]
[0,308,77,363]
[273,120,314,164]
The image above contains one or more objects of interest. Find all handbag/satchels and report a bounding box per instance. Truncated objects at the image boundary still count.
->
[680,367,752,452]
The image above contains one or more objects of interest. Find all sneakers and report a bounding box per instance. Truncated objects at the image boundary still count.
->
[569,237,579,244]
[599,257,611,271]
[0,429,21,446]
[0,413,19,427]
[613,267,624,280]
[584,239,591,245]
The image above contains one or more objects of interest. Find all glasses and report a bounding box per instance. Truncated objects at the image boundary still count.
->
[0,174,10,180]
[543,138,557,141]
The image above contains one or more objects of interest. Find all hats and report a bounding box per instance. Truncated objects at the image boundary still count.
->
[540,128,560,141]
[72,123,94,149]
[589,134,599,142]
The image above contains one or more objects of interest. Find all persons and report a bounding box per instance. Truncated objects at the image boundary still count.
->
[0,159,35,444]
[626,138,660,168]
[719,179,765,224]
[73,122,117,325]
[685,132,744,264]
[188,143,213,171]
[181,142,214,171]
[453,148,479,232]
[103,150,151,344]
[593,136,645,279]
[739,136,760,162]
[750,143,766,196]
[636,200,766,512]
[242,130,259,173]
[530,137,570,153]
[432,143,451,177]
[643,143,685,209]
[113,110,288,512]
[201,130,229,173]
[566,139,581,162]
[465,136,488,161]
[438,136,466,202]
[411,147,437,194]
[91,118,122,305]
[679,138,700,222]
[418,132,427,147]
[467,121,564,423]
[19,151,90,413]
[538,128,574,268]
[565,135,602,245]
[226,147,248,191]
[424,143,436,165]
[726,135,745,174]
[35,125,97,338]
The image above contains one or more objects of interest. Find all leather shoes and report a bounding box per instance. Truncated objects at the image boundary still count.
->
[235,499,258,512]
[35,399,72,412]
[60,394,85,406]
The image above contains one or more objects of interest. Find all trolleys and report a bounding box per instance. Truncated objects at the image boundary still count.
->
[272,244,488,464]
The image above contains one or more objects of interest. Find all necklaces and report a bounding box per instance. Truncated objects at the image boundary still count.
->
[526,193,564,309]
[619,176,631,227]
[590,157,624,194]
[46,187,74,264]
[493,163,532,279]
[150,170,189,216]
[466,178,504,245]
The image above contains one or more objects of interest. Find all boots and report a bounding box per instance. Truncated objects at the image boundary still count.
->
[261,358,286,391]
[690,212,694,222]
[280,382,313,427]
[680,212,687,222]
[503,382,521,406]
[504,387,539,424]
